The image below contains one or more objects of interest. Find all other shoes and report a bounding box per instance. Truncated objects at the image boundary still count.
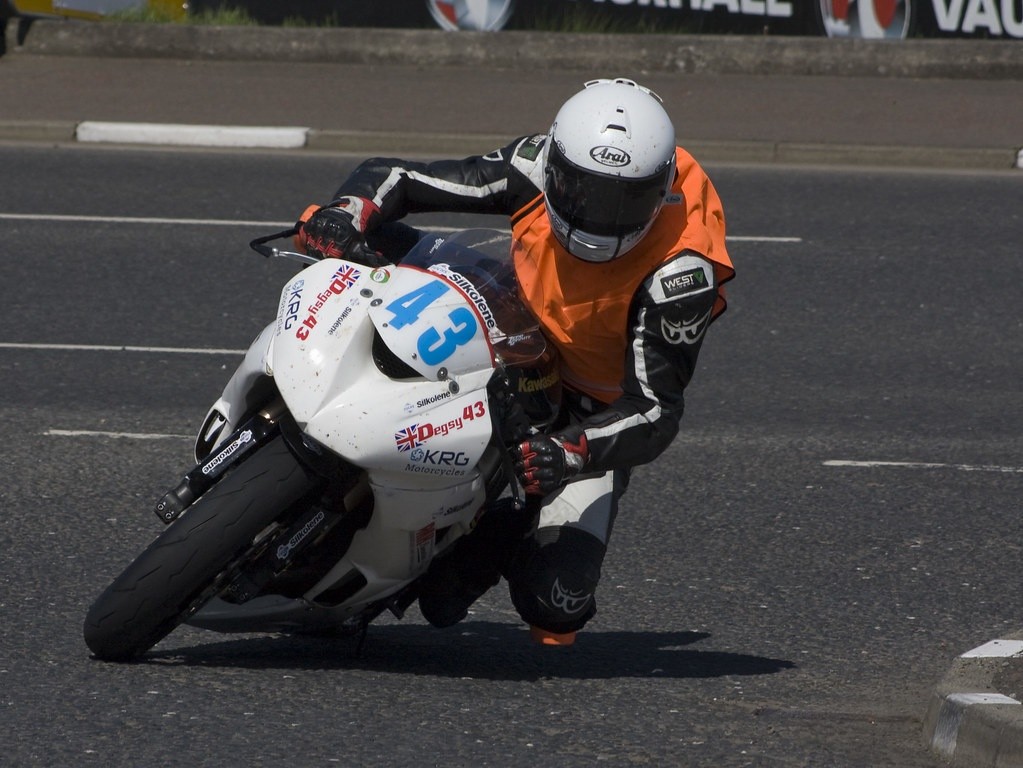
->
[420,497,525,627]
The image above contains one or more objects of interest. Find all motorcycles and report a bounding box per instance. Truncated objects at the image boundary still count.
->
[84,229,561,660]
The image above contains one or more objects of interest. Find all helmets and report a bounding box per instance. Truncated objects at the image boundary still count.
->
[541,77,675,264]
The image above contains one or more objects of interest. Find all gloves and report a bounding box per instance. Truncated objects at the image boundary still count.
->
[304,192,382,260]
[515,425,591,497]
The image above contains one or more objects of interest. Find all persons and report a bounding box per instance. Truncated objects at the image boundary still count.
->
[295,79,736,644]
